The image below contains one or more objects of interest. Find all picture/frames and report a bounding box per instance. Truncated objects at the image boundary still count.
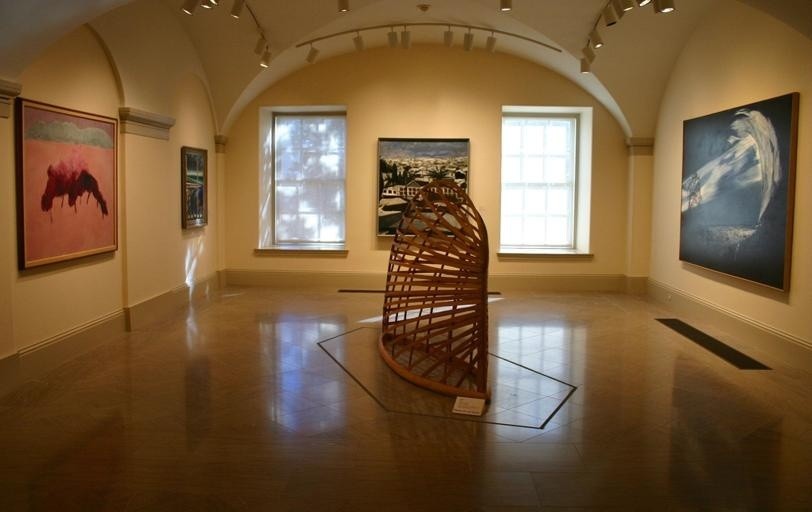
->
[180,144,209,230]
[375,135,473,241]
[13,94,119,274]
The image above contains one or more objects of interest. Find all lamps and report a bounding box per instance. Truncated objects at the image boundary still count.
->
[293,21,499,64]
[180,0,272,68]
[336,0,350,13]
[579,0,675,73]
[499,0,512,12]
